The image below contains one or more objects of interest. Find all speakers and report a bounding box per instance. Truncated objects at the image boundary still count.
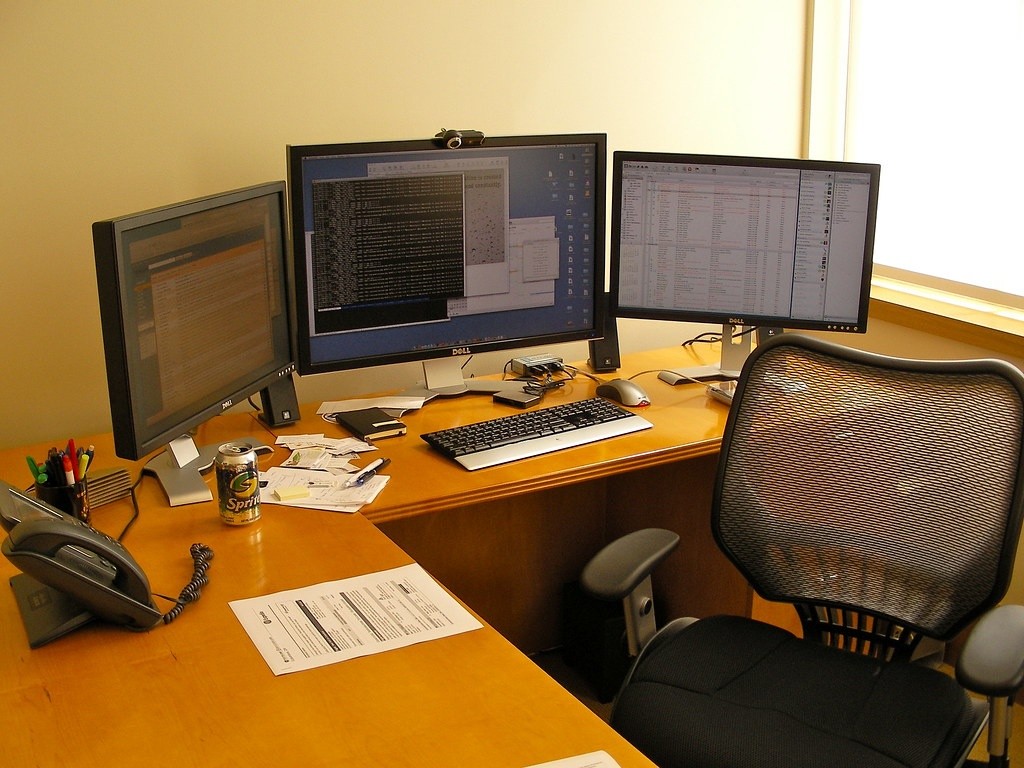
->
[587,292,621,374]
[258,372,301,427]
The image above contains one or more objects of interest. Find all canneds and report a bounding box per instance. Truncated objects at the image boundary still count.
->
[214,442,262,525]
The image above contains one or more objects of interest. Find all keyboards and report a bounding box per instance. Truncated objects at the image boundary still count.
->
[419,397,654,470]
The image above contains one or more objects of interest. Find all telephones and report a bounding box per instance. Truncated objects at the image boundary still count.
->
[0,478,162,633]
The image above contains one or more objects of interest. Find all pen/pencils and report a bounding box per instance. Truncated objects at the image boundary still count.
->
[346,457,391,490]
[26,438,95,487]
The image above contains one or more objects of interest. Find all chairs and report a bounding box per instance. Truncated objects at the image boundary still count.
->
[579,329,1024,767]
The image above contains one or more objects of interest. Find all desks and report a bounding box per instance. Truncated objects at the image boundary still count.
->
[249,337,887,703]
[0,412,658,768]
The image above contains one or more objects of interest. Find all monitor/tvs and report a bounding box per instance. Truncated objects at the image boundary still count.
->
[285,133,607,419]
[92,179,299,507]
[610,151,881,394]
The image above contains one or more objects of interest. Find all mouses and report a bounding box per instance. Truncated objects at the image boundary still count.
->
[596,378,651,407]
